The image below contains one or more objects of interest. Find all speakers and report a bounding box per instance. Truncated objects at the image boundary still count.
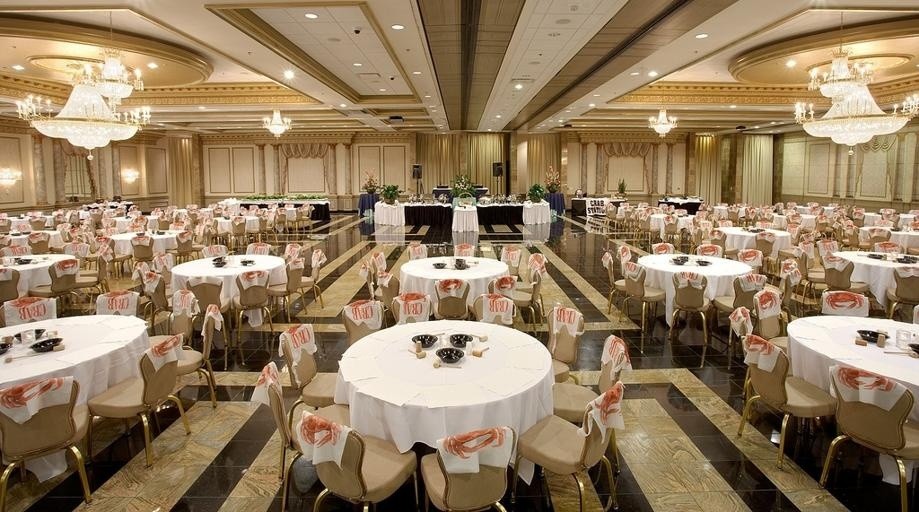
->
[493,162,503,176]
[413,164,423,179]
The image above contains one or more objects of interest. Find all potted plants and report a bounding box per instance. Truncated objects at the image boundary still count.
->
[614,177,628,198]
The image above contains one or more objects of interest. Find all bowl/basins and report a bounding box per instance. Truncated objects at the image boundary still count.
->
[742,226,765,233]
[4,231,31,236]
[0,327,65,356]
[224,216,230,220]
[136,230,165,238]
[890,227,900,231]
[909,342,919,352]
[436,348,463,362]
[13,257,33,264]
[211,255,255,267]
[866,252,918,265]
[858,329,890,343]
[432,258,468,269]
[450,333,473,347]
[411,333,438,348]
[671,256,711,265]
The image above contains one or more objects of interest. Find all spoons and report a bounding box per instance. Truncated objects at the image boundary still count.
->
[472,348,489,358]
[884,350,919,358]
[3,354,37,363]
[470,334,488,343]
[432,362,462,369]
[408,349,426,359]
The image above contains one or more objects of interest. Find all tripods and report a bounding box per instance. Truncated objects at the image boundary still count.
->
[493,176,501,199]
[413,178,422,202]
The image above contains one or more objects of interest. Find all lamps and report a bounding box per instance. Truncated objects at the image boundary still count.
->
[84,8,151,108]
[790,51,919,157]
[261,105,295,140]
[643,105,683,138]
[803,10,875,103]
[12,61,155,159]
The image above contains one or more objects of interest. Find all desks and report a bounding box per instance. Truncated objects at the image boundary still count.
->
[786,316,919,488]
[335,320,555,487]
[0,315,149,483]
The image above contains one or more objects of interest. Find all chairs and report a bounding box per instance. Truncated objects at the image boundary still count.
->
[174,304,219,408]
[1,376,92,510]
[421,426,519,511]
[552,334,632,474]
[251,362,350,479]
[1,185,919,373]
[738,334,838,469]
[87,334,192,468]
[819,365,919,510]
[511,380,624,510]
[278,324,340,433]
[729,306,760,423]
[282,403,419,511]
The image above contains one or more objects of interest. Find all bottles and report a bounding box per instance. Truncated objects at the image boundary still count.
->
[855,338,867,346]
[415,341,422,352]
[877,334,885,348]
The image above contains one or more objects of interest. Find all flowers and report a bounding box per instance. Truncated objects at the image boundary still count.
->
[363,167,381,190]
[542,164,562,189]
[449,175,476,197]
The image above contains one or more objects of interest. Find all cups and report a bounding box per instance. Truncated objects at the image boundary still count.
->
[894,328,910,348]
[2,258,10,267]
[439,335,449,349]
[465,336,480,355]
